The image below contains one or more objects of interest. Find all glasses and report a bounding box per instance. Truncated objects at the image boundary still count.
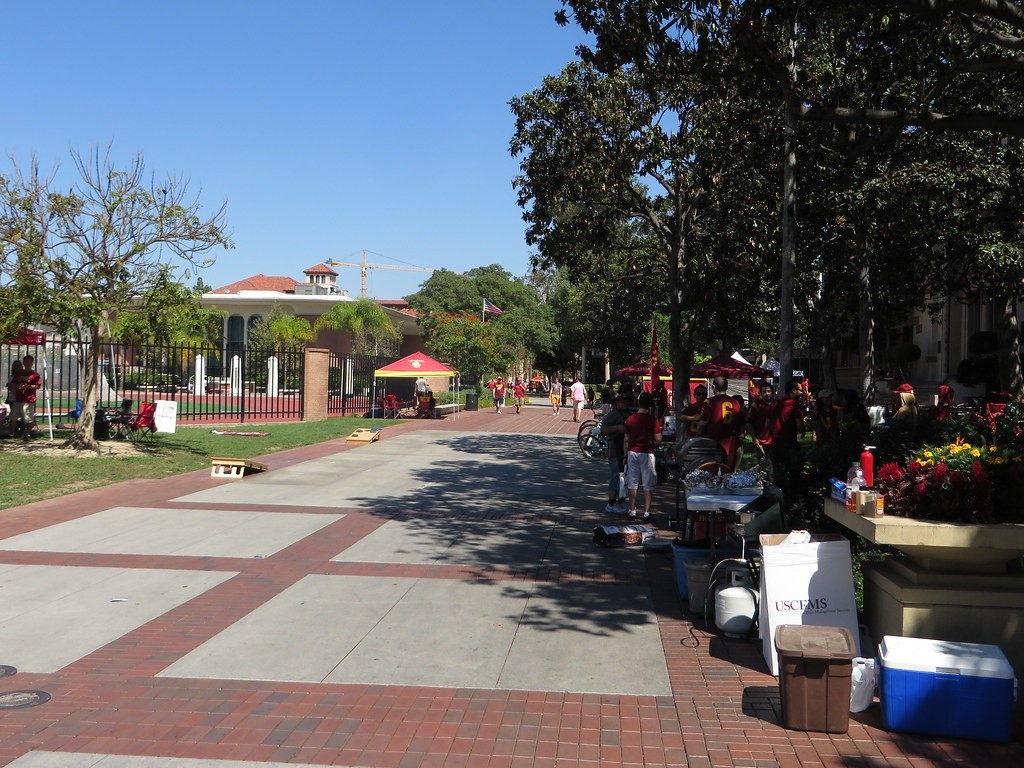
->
[792,387,803,392]
[695,392,706,396]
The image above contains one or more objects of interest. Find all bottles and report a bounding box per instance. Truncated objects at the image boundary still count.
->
[865,486,884,518]
[846,462,864,510]
[672,429,676,442]
[717,467,722,488]
[851,471,866,512]
[856,486,870,514]
[664,422,671,431]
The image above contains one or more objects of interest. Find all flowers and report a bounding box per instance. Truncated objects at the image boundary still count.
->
[884,435,1024,522]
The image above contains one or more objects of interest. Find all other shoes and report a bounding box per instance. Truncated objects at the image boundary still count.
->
[0,434,15,439]
[24,434,30,441]
[497,409,500,415]
[516,409,520,413]
[557,411,559,415]
[628,509,637,517]
[573,416,579,422]
[644,512,652,517]
[553,412,556,416]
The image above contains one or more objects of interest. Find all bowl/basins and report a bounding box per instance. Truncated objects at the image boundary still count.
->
[704,477,722,488]
[682,480,701,489]
[645,540,671,551]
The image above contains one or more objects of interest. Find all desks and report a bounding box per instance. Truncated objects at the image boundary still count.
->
[683,488,761,549]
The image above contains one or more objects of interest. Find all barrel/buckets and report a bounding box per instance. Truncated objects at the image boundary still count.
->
[684,558,747,615]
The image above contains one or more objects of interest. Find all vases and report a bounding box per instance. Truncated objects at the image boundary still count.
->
[822,497,1024,673]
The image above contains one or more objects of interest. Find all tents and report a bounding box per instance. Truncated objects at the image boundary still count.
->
[616,360,670,376]
[3,327,53,441]
[372,351,459,421]
[691,354,774,379]
[760,357,804,381]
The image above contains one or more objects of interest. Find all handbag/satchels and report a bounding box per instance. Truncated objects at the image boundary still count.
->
[618,463,629,498]
[5,396,13,404]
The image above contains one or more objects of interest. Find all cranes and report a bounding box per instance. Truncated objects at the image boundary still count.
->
[323,248,433,300]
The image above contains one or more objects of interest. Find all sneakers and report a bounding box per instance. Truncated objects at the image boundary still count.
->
[606,503,627,514]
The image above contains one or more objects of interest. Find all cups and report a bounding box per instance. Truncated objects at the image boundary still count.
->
[662,431,672,442]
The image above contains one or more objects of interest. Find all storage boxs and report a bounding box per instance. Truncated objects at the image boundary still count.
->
[774,625,855,735]
[760,534,862,676]
[670,538,748,615]
[879,635,1019,745]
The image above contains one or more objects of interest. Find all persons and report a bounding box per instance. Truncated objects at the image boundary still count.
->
[601,380,668,522]
[549,377,562,416]
[7,355,42,438]
[891,383,917,421]
[524,381,544,397]
[680,375,806,471]
[588,387,595,403]
[487,379,496,391]
[414,377,436,417]
[811,388,871,444]
[939,380,955,406]
[512,376,526,413]
[492,376,506,414]
[571,377,586,423]
[508,381,514,390]
[802,378,809,393]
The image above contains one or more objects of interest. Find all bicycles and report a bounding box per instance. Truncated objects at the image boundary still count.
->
[576,411,610,462]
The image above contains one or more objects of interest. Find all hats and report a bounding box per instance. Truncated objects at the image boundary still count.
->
[517,377,521,381]
[638,392,652,405]
[614,394,628,401]
[818,389,832,399]
[498,376,502,380]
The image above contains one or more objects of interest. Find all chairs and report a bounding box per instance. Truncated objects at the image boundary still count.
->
[68,398,157,443]
[382,394,437,420]
